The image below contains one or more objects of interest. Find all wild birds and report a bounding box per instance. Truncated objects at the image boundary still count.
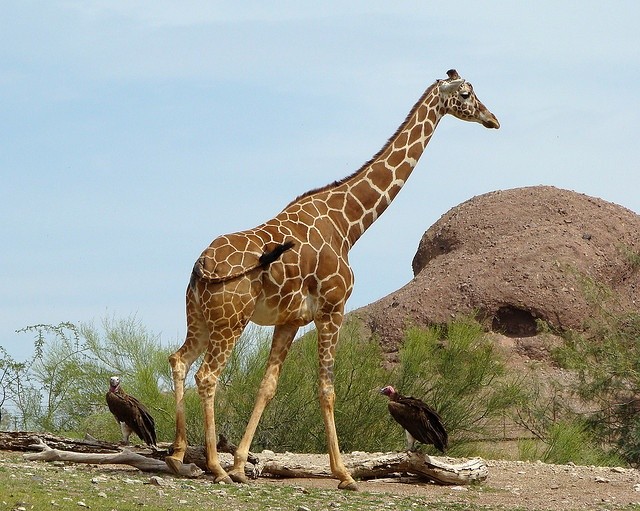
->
[107,376,156,448]
[378,386,448,455]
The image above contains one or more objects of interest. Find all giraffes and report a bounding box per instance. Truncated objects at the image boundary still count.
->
[166,69,501,489]
